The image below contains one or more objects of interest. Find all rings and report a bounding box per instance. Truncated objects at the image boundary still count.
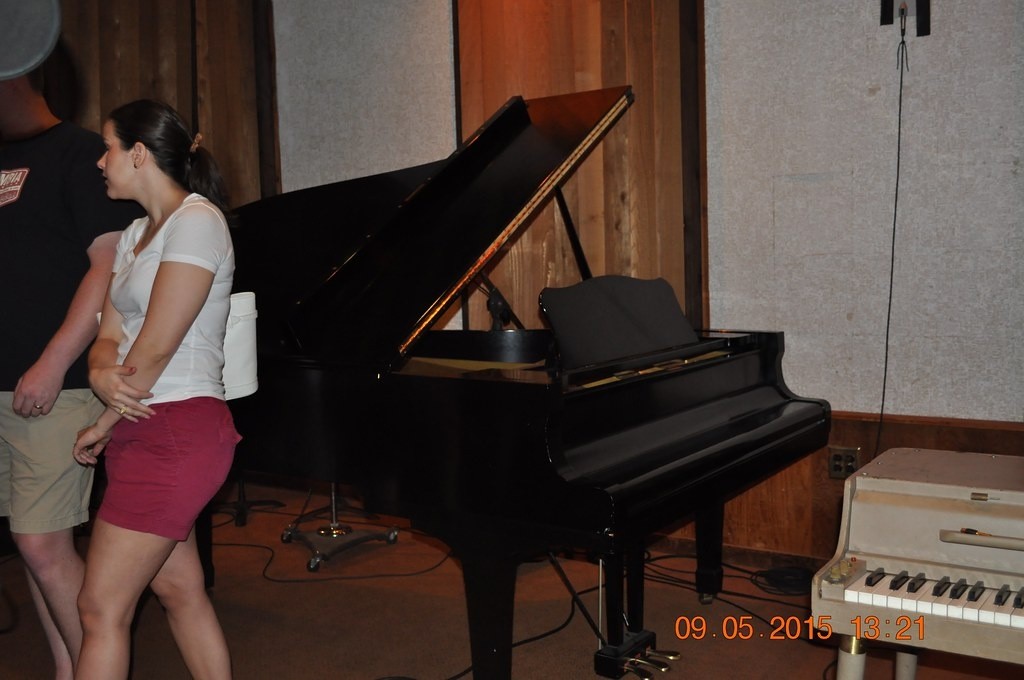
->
[32,404,43,409]
[120,406,128,415]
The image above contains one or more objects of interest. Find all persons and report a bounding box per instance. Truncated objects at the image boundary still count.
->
[69,99,242,679]
[0,65,133,679]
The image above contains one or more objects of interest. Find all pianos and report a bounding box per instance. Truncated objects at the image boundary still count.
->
[194,82,834,680]
[810,445,1024,680]
